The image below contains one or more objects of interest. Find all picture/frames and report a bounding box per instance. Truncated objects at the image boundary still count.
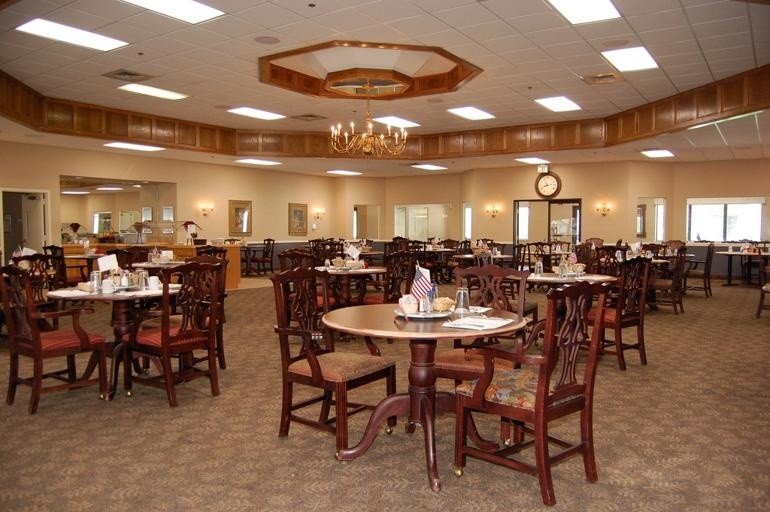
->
[287,202,309,237]
[163,206,174,221]
[635,204,647,238]
[227,199,254,237]
[141,207,152,221]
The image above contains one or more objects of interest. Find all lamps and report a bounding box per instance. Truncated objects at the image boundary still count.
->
[313,206,326,219]
[327,76,410,158]
[198,201,216,216]
[596,203,613,217]
[486,205,499,218]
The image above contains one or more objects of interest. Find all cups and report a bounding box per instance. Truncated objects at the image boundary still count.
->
[614,247,679,261]
[322,259,365,270]
[533,256,588,280]
[87,266,161,295]
[391,285,472,321]
[425,241,562,256]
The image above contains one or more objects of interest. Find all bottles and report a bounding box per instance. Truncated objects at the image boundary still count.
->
[729,244,760,254]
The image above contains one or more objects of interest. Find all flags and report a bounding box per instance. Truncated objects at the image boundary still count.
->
[410,264,433,302]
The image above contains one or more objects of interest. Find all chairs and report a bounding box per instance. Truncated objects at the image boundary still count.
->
[453,280,612,506]
[269,265,397,459]
[756,249,770,317]
[269,231,714,371]
[0,232,241,415]
[224,238,275,278]
[715,239,770,288]
[434,264,531,441]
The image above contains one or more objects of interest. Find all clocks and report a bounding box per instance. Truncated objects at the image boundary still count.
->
[534,171,562,200]
